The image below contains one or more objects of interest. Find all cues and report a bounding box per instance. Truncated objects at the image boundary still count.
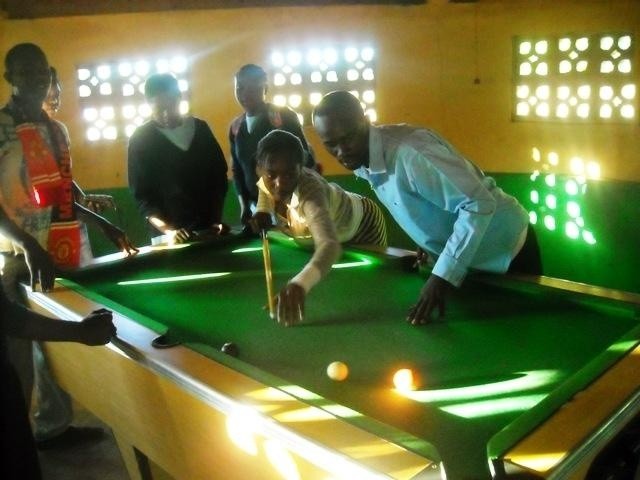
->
[260,226,277,318]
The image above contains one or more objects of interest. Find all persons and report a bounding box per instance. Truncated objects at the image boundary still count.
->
[247,130,388,328]
[228,62,317,226]
[126,71,230,244]
[315,90,542,327]
[43,67,116,262]
[0,293,118,480]
[1,42,140,449]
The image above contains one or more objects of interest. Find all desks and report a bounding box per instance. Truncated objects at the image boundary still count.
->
[12,226,639,480]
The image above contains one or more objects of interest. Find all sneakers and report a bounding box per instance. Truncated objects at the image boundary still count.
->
[34,423,105,454]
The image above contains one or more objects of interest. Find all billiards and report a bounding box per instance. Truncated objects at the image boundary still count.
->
[392,369,419,391]
[327,360,349,382]
